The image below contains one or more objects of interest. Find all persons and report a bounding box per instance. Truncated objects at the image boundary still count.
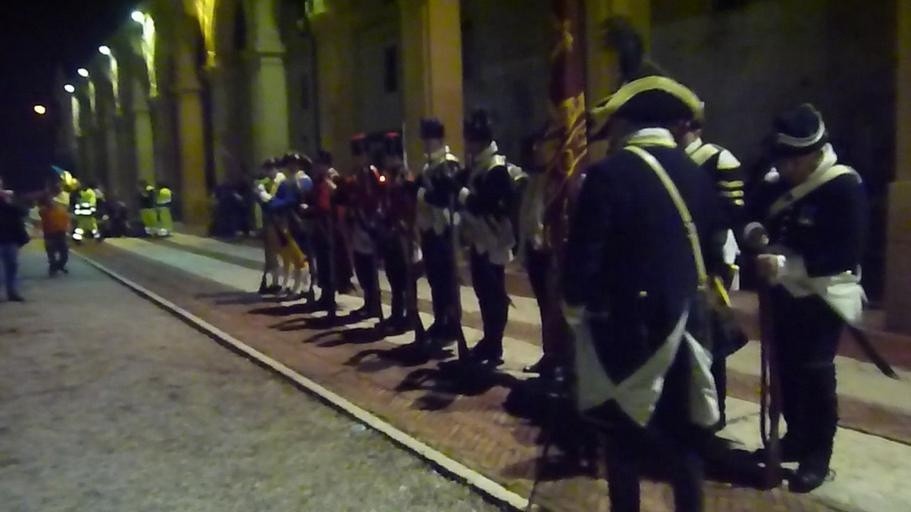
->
[736,104,871,490]
[253,107,529,371]
[1,174,174,302]
[515,65,746,512]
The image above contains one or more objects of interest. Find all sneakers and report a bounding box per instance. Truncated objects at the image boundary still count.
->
[788,463,828,493]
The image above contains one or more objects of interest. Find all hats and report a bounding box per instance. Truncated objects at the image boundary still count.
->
[587,73,706,141]
[775,104,830,159]
